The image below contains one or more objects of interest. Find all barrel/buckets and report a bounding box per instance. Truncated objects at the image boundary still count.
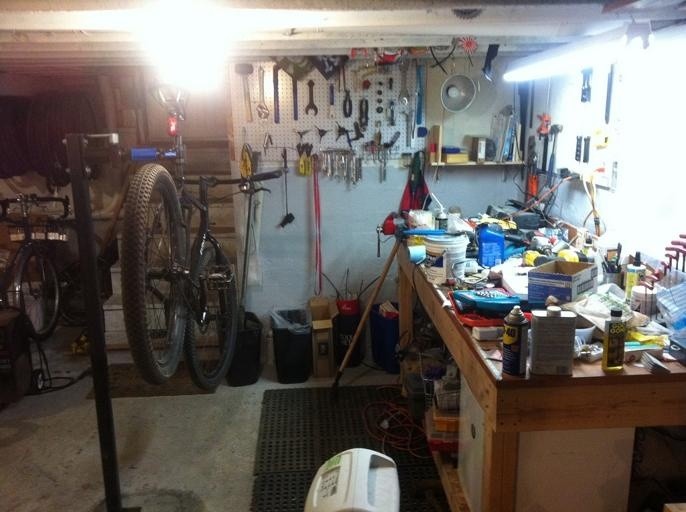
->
[423,234,470,286]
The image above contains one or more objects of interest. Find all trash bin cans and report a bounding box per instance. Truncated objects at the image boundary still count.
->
[218,312,263,385]
[270,309,314,383]
[370,301,400,372]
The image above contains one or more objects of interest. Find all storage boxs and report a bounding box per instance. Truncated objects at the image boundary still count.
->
[526,259,601,308]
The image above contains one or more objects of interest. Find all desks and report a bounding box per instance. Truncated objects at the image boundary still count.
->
[386,210,686,512]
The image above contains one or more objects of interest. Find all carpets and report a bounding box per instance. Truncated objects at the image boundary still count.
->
[249,383,449,510]
[83,356,220,402]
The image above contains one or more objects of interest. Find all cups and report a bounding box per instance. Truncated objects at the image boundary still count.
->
[443,249,466,284]
[336,298,359,315]
[412,236,425,245]
[602,271,621,287]
[407,245,426,262]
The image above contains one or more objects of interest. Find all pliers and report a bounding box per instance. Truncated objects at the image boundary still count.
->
[343,90,353,119]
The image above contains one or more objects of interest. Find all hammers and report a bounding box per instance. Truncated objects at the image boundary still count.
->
[235,62,254,124]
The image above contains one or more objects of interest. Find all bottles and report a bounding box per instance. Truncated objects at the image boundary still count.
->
[602,308,627,371]
[502,305,528,376]
[492,258,503,279]
[523,236,597,267]
[528,306,578,377]
[435,211,448,230]
[477,221,505,268]
[625,250,646,306]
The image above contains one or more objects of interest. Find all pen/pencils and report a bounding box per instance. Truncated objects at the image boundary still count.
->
[601,255,622,273]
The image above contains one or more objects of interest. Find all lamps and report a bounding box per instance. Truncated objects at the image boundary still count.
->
[438,72,475,114]
[501,16,656,87]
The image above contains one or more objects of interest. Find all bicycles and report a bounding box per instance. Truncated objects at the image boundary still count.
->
[121,161,285,391]
[0,194,71,340]
